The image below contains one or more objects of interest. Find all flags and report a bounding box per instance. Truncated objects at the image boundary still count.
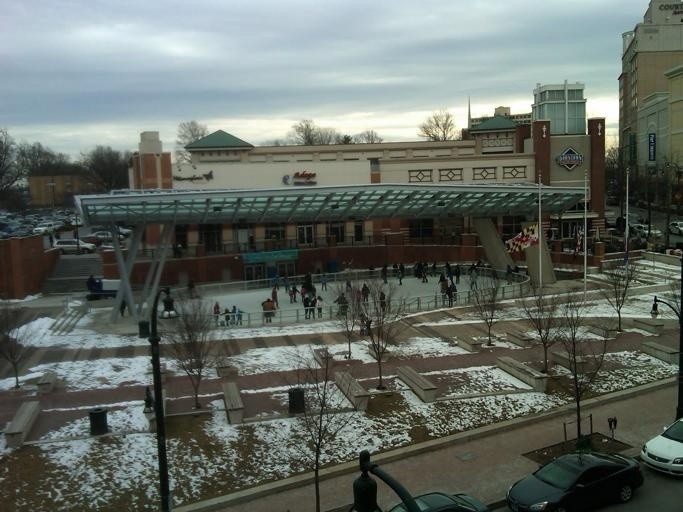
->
[504,222,539,253]
[576,224,584,252]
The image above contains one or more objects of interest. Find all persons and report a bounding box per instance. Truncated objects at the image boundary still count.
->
[333,267,390,316]
[214,301,246,325]
[413,260,519,308]
[393,262,404,285]
[262,271,327,322]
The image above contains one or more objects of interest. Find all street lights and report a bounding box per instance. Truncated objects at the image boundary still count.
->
[648,296,682,421]
[665,162,679,187]
[142,284,183,512]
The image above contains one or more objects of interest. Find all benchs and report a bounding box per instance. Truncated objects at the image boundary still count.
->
[222,382,244,424]
[497,356,551,390]
[592,324,617,337]
[368,344,390,360]
[313,349,333,366]
[552,351,588,372]
[457,335,482,352]
[158,363,167,383]
[5,400,40,447]
[216,356,230,377]
[507,331,530,346]
[335,372,371,410]
[37,372,58,393]
[633,319,664,334]
[641,341,679,363]
[395,365,438,402]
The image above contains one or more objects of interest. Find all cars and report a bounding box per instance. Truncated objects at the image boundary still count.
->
[639,417,683,478]
[622,212,662,239]
[504,449,644,512]
[605,185,619,206]
[385,490,489,511]
[666,221,682,235]
[0,206,132,254]
